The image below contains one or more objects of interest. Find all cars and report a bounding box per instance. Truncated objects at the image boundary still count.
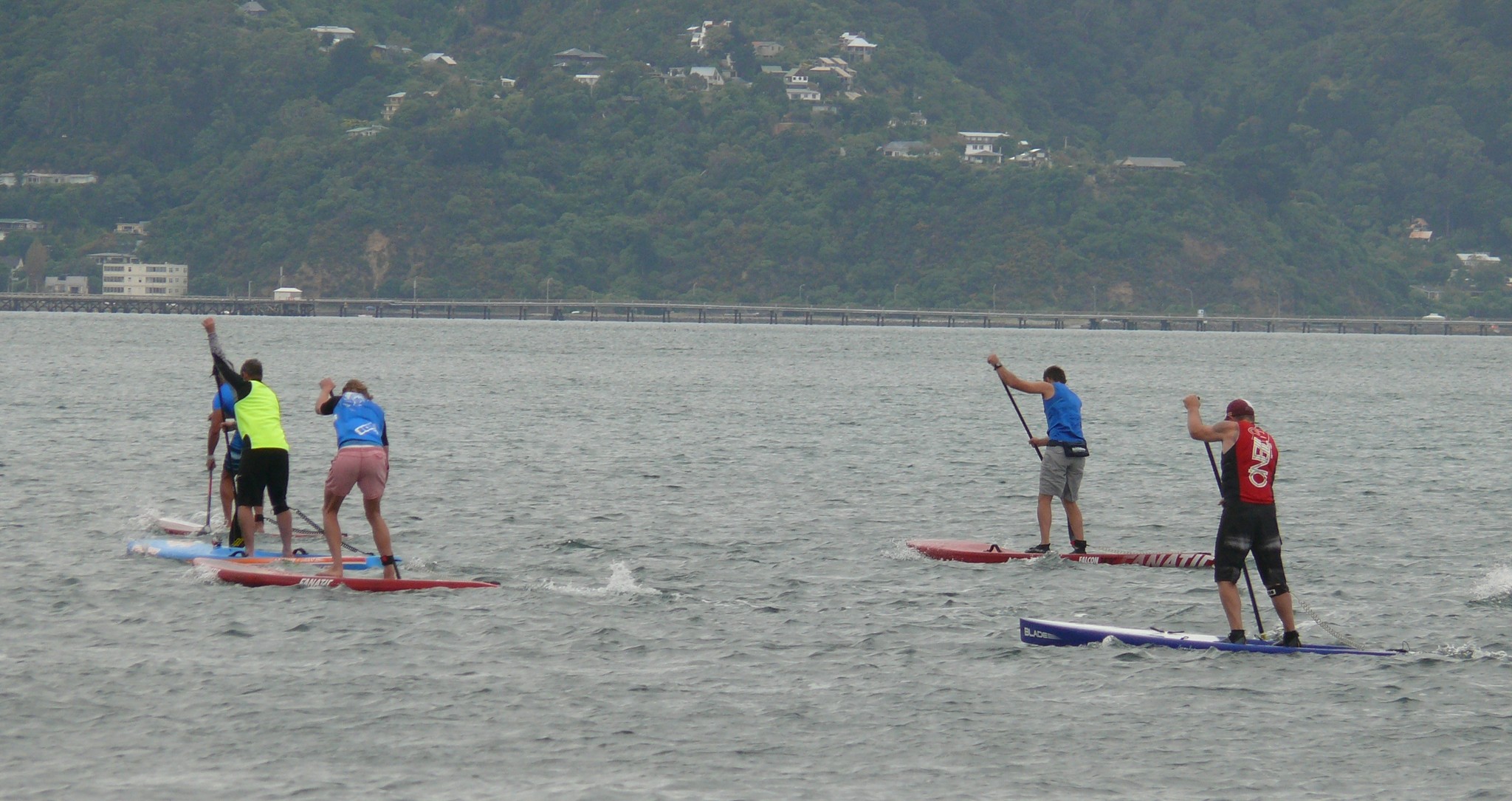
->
[366,306,377,311]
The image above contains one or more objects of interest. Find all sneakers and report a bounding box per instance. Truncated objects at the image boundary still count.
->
[1024,543,1050,553]
[1213,632,1246,644]
[1272,639,1302,648]
[1069,549,1086,554]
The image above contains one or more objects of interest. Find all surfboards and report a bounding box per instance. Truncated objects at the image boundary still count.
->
[906,539,1218,567]
[1017,614,1411,657]
[125,538,402,572]
[158,516,348,541]
[192,557,502,591]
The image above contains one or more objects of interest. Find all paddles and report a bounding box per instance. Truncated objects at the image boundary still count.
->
[998,374,1074,549]
[329,390,403,579]
[1195,396,1267,639]
[213,369,246,548]
[199,469,213,538]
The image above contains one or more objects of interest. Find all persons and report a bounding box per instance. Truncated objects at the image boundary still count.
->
[314,376,399,582]
[1183,394,1302,648]
[987,354,1088,554]
[198,317,298,561]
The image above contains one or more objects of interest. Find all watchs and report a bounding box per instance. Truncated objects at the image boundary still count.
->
[994,363,1002,369]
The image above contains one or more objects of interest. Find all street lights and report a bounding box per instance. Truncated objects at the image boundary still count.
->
[994,284,996,313]
[693,283,697,298]
[1092,286,1095,313]
[894,284,899,301]
[1185,288,1192,309]
[547,277,554,315]
[35,280,38,293]
[249,281,252,300]
[800,284,805,301]
[414,276,418,301]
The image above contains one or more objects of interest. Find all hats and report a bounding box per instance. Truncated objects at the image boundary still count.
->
[1224,399,1254,420]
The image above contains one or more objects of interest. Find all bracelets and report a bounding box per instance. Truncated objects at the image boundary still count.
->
[207,455,214,459]
